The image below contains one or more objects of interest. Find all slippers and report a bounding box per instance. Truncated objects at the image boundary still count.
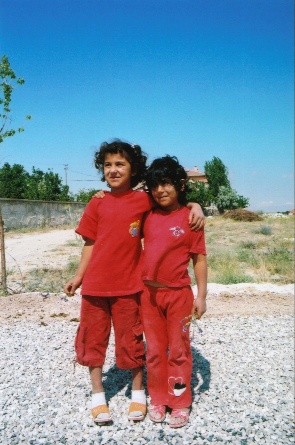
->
[128,400,148,421]
[88,398,113,426]
[150,400,164,421]
[168,405,193,427]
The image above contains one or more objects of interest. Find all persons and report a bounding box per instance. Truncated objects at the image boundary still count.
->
[92,153,207,429]
[63,136,205,422]
